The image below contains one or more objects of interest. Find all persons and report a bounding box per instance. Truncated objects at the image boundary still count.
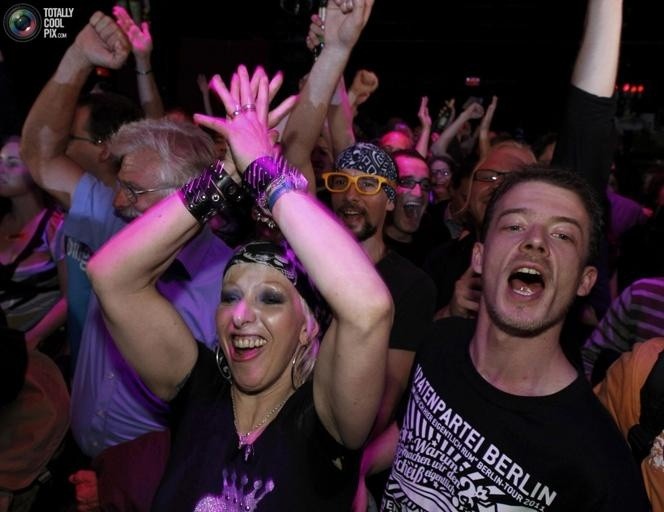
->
[111,5,165,120]
[478,95,499,158]
[85,66,395,510]
[359,93,430,161]
[438,93,482,151]
[539,139,556,166]
[198,73,214,119]
[607,82,664,250]
[280,0,438,439]
[0,134,67,511]
[305,3,430,265]
[641,158,661,278]
[64,64,111,169]
[425,154,457,213]
[430,0,622,327]
[350,160,652,512]
[581,277,664,383]
[432,104,484,154]
[593,337,664,512]
[1,308,98,511]
[23,12,238,512]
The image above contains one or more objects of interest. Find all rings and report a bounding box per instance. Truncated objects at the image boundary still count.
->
[229,109,242,120]
[242,104,255,112]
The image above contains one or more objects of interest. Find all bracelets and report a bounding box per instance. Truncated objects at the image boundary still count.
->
[264,182,295,209]
[136,70,152,76]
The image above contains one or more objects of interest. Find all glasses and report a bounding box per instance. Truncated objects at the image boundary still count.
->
[116,178,164,205]
[321,172,398,196]
[472,167,513,182]
[431,168,450,177]
[398,176,434,192]
[68,134,99,145]
